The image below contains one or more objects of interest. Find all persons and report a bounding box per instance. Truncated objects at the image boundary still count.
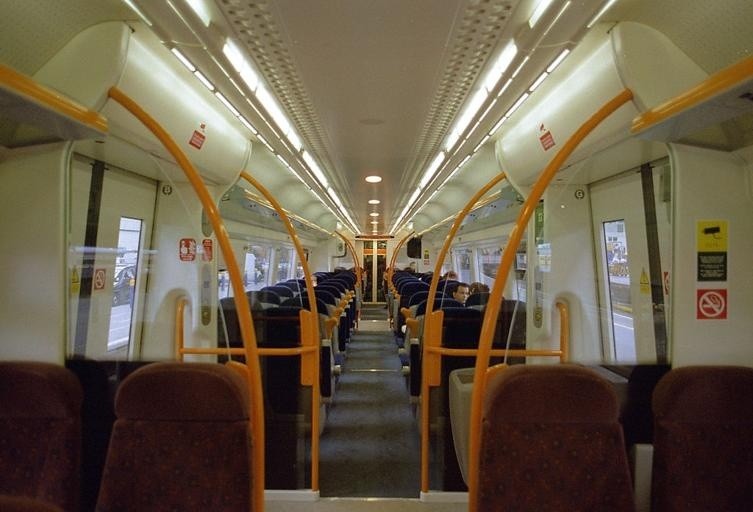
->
[405,262,416,272]
[444,273,456,280]
[453,283,470,306]
[471,282,483,292]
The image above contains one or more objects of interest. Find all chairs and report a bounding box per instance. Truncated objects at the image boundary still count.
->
[0,360,84,512]
[650,365,752,512]
[92,360,256,512]
[476,363,637,512]
[217,270,358,436]
[391,271,526,439]
